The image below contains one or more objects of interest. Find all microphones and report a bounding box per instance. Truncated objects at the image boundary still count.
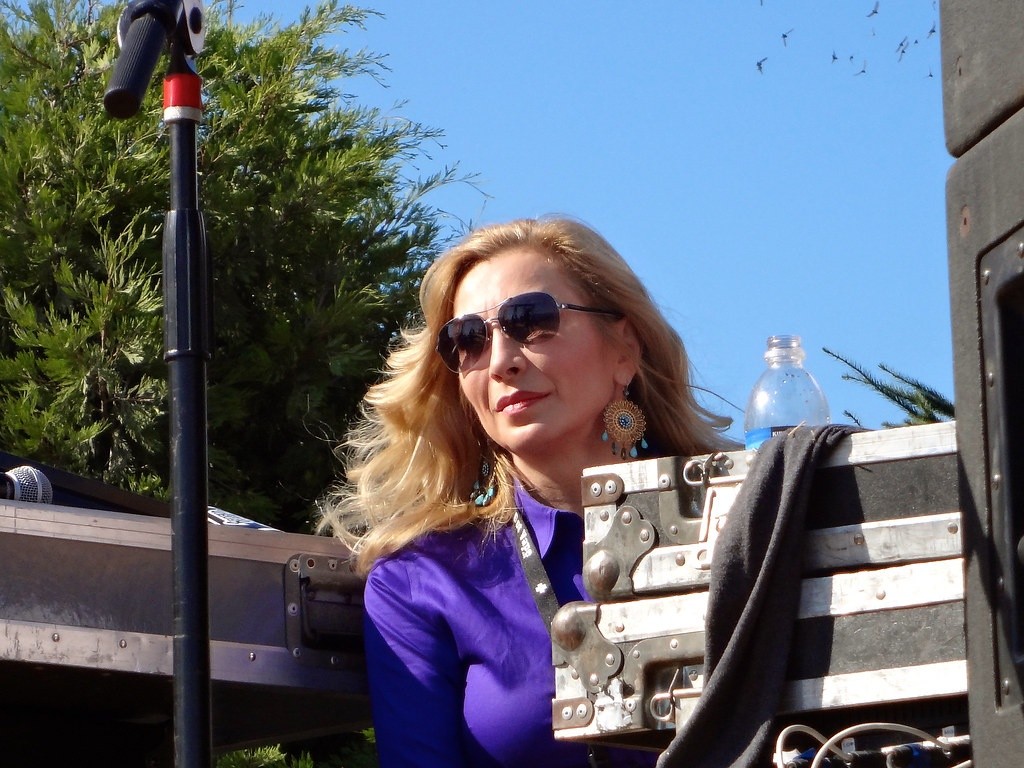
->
[0,465,54,503]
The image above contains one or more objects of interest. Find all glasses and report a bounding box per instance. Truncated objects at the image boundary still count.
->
[435,291,621,373]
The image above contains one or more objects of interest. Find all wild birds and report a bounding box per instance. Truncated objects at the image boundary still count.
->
[866,1,880,18]
[756,57,768,73]
[781,28,794,47]
[871,0,937,79]
[853,60,867,76]
[849,55,854,62]
[831,50,838,63]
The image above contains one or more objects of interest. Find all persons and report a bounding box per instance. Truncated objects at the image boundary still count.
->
[317,216,749,768]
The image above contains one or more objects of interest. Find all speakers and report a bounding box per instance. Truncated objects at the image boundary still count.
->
[944,0,1024,768]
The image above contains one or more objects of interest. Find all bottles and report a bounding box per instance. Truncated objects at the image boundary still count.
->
[741,333,831,452]
